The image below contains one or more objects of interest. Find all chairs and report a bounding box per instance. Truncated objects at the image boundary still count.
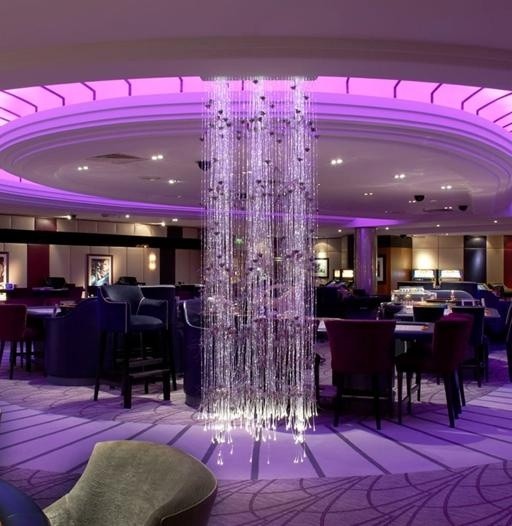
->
[406,312,473,415]
[411,277,436,286]
[437,275,464,282]
[452,306,486,388]
[92,285,171,410]
[413,305,444,323]
[440,282,478,298]
[397,281,434,291]
[324,319,396,432]
[315,284,349,318]
[397,314,472,427]
[0,304,35,379]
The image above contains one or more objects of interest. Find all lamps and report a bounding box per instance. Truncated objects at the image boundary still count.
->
[332,268,354,280]
[148,250,156,272]
[438,269,462,279]
[67,215,77,220]
[414,195,425,201]
[458,206,468,211]
[194,75,322,468]
[411,269,435,280]
[198,160,212,172]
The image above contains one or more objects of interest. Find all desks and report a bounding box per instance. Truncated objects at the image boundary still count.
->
[0,285,84,306]
[379,299,486,344]
[20,304,63,371]
[394,322,434,426]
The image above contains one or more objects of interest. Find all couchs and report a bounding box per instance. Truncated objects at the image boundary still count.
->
[42,440,218,526]
[181,298,257,411]
[42,297,108,378]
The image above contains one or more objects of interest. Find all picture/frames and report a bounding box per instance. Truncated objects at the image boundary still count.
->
[378,254,386,284]
[86,253,114,292]
[0,251,9,303]
[315,258,329,278]
